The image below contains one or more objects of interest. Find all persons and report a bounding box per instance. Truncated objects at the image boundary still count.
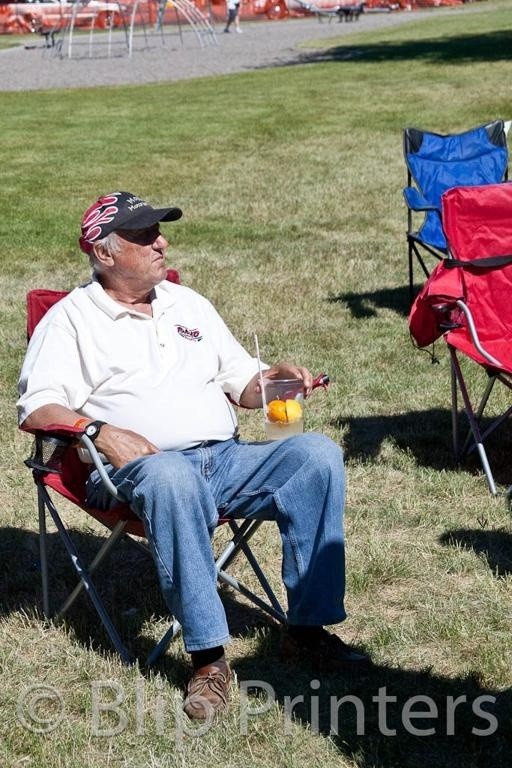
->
[221,0,244,33]
[14,189,378,724]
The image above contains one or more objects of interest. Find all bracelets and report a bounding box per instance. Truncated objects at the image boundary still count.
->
[70,416,91,427]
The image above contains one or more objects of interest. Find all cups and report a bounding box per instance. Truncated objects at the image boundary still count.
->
[258,378,304,441]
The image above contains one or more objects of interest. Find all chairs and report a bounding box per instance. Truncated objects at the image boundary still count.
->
[23,269,288,676]
[404,119,508,305]
[406,181,512,496]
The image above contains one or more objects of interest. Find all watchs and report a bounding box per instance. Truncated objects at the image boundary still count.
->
[83,419,107,441]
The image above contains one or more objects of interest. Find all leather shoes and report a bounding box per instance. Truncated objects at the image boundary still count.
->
[184,664,230,721]
[280,628,372,679]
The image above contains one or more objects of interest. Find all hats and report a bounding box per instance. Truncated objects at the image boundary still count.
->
[79,192,183,254]
